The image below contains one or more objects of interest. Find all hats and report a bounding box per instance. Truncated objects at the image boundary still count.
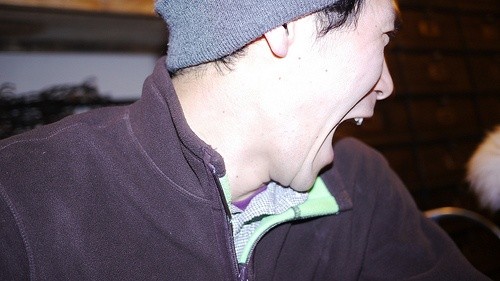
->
[154,0,340,72]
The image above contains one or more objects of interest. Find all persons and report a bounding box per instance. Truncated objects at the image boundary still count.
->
[1,1,495,281]
